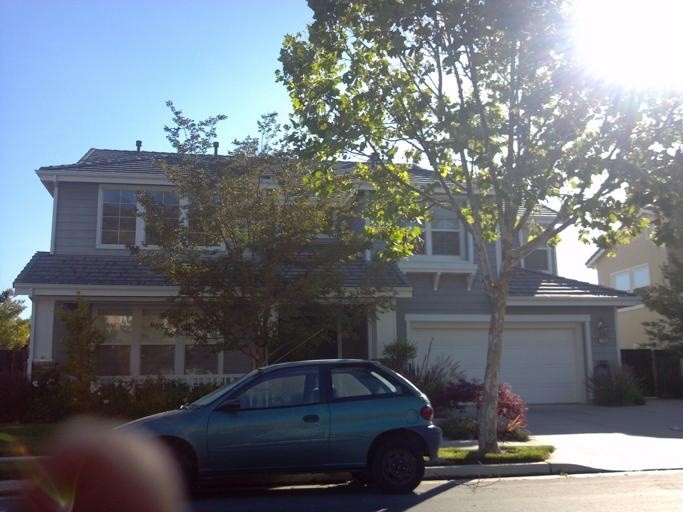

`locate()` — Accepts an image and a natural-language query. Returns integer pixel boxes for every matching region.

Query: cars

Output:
[105,357,441,494]
